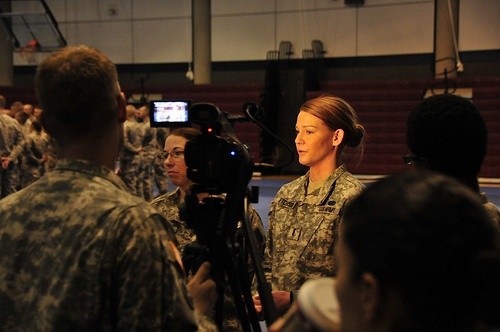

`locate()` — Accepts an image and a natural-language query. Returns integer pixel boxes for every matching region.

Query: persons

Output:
[0,45,267,332]
[332,94,500,332]
[253,94,367,332]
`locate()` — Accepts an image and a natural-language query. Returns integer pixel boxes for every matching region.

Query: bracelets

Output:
[290,291,294,303]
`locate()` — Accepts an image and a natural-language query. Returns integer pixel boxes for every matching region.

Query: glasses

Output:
[403,153,427,168]
[158,149,184,164]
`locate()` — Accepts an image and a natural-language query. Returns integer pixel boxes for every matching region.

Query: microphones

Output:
[242,100,266,123]
